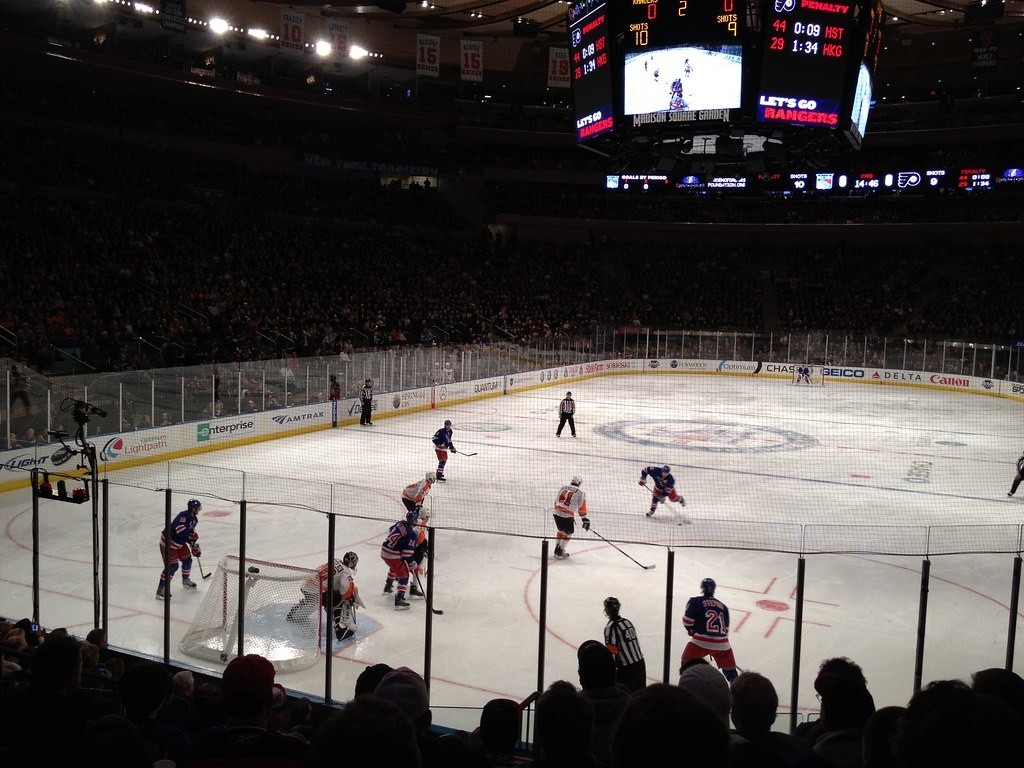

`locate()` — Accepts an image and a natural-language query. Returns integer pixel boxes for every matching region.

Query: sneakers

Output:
[436,475,446,483]
[182,577,197,589]
[408,582,425,600]
[155,587,172,601]
[382,587,393,596]
[553,546,569,559]
[394,593,411,610]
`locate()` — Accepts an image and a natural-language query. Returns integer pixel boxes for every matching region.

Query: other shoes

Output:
[679,496,685,507]
[646,510,654,517]
[1007,491,1013,497]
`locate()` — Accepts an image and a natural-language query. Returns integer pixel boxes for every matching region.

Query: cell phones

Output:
[32,624,39,631]
[98,663,106,675]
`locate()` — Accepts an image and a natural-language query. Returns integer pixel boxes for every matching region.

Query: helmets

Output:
[406,506,430,524]
[661,465,671,473]
[700,578,716,595]
[426,471,436,480]
[343,551,359,570]
[444,419,452,427]
[365,378,371,382]
[570,472,584,485]
[566,391,572,396]
[602,597,621,619]
[187,498,201,508]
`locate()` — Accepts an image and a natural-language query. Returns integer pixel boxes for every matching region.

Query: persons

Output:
[1006,450,1024,497]
[797,365,812,385]
[0,292,1024,768]
[642,53,693,110]
[682,579,739,682]
[409,509,429,595]
[155,499,202,598]
[330,375,340,400]
[359,378,373,427]
[432,420,456,480]
[556,392,577,437]
[639,465,685,517]
[381,511,422,609]
[605,597,647,690]
[402,473,437,521]
[286,551,358,641]
[553,477,590,560]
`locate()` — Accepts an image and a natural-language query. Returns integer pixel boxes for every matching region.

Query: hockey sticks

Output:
[447,447,477,457]
[416,573,443,614]
[588,527,656,569]
[197,556,212,580]
[644,484,693,525]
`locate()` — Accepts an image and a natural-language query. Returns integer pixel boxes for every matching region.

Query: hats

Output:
[678,657,731,717]
[372,671,427,723]
[576,639,615,689]
[222,655,276,704]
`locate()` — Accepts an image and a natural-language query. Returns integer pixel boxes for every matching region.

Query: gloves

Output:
[449,446,456,453]
[638,479,647,486]
[582,518,590,532]
[660,497,666,504]
[189,532,202,558]
[409,561,419,575]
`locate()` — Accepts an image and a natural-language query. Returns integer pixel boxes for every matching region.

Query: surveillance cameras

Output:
[91,407,107,418]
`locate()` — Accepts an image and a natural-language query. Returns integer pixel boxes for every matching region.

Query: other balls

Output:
[678,524,682,526]
